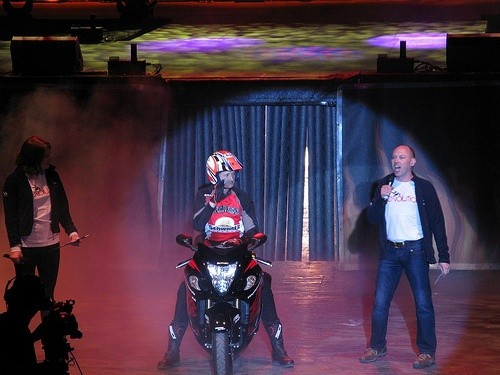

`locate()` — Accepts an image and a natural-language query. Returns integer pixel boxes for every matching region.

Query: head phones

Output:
[4,274,55,312]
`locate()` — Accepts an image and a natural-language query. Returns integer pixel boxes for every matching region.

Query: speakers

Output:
[10,36,84,75]
[446,33,500,71]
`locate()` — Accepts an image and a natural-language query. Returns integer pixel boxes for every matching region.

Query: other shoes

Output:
[358,348,388,363]
[413,354,436,369]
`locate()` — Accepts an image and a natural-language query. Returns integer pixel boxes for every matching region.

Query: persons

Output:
[359,145,451,369]
[2,136,81,352]
[0,273,71,375]
[158,151,294,369]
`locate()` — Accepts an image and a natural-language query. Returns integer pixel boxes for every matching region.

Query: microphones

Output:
[3,254,29,264]
[380,176,394,207]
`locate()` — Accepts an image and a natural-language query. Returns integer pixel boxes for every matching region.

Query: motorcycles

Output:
[175,233,273,375]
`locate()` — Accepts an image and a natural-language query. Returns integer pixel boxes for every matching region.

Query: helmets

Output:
[206,150,243,185]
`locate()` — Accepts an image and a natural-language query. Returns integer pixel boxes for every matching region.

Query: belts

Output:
[387,240,411,249]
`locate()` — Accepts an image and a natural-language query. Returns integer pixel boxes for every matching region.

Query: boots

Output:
[266,318,296,368]
[157,324,186,369]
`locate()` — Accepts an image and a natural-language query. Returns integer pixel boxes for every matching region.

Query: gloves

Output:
[254,233,266,242]
[209,180,232,203]
